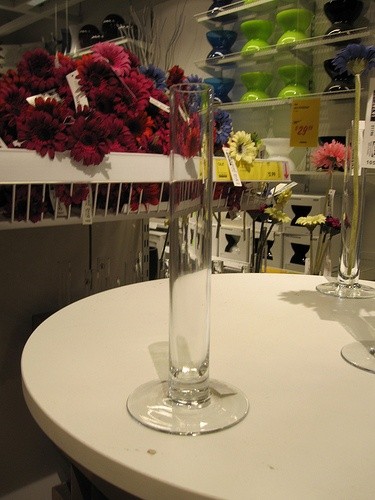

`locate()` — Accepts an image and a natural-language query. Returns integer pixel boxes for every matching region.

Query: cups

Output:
[315,130,374,299]
[340,337,375,373]
[126,84,249,436]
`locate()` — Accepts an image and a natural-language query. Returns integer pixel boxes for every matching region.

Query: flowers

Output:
[0,42,269,222]
[253,140,352,273]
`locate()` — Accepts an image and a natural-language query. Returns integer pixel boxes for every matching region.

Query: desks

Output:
[19,268,375,500]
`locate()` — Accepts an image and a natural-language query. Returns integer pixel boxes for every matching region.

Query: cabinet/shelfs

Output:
[194,0,375,272]
[0,0,153,67]
